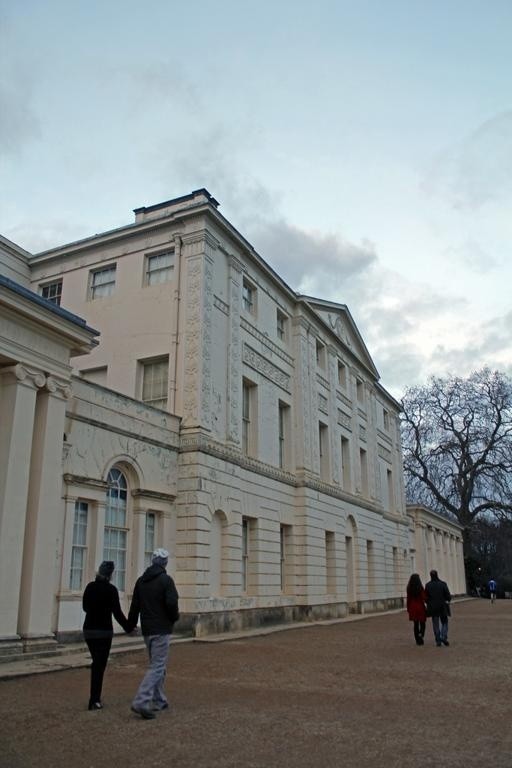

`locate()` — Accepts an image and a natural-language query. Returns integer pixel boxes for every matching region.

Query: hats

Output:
[97,559,117,579]
[150,547,170,568]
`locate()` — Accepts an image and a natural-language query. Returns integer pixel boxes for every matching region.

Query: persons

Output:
[469,584,487,598]
[80,559,126,711]
[424,570,454,645]
[487,576,498,603]
[125,547,179,720]
[404,572,429,645]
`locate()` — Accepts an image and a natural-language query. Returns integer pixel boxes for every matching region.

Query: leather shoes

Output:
[153,702,169,712]
[130,705,156,720]
[414,633,449,647]
[88,700,104,711]
